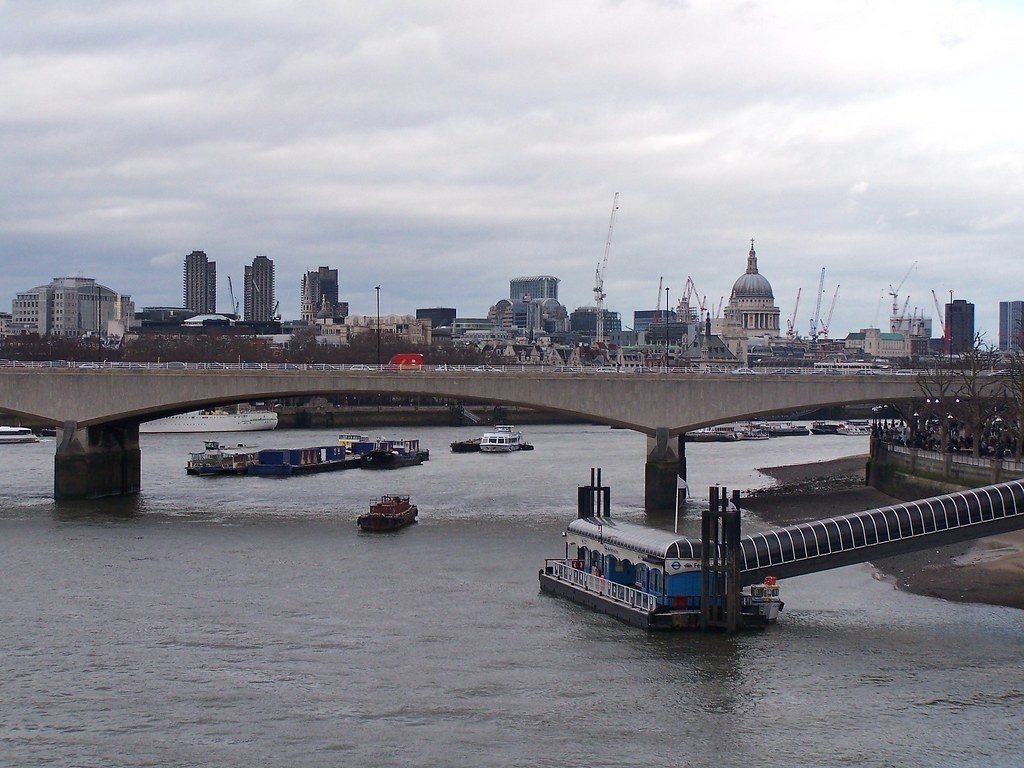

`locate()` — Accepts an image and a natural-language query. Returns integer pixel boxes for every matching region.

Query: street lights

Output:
[949,290,954,376]
[665,286,671,372]
[375,284,381,369]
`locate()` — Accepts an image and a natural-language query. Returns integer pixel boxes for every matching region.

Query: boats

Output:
[684,420,903,442]
[449,438,482,452]
[478,425,522,453]
[184,432,431,476]
[537,467,785,632]
[139,407,279,433]
[356,494,418,531]
[0,425,39,448]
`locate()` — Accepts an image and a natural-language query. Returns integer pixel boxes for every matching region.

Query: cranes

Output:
[652,276,663,322]
[931,288,946,333]
[887,260,919,335]
[785,287,803,338]
[591,191,623,342]
[807,267,826,340]
[677,275,724,321]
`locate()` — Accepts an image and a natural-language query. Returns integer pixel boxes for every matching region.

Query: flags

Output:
[678,477,690,498]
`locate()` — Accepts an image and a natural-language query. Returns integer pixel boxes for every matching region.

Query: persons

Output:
[870,423,1015,459]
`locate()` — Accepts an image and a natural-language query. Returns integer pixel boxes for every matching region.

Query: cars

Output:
[0,358,376,371]
[989,369,1021,376]
[434,364,502,373]
[553,366,917,376]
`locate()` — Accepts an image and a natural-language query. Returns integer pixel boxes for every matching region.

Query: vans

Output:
[382,354,424,371]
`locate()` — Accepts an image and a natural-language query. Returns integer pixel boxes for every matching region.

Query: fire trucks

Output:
[818,284,840,339]
